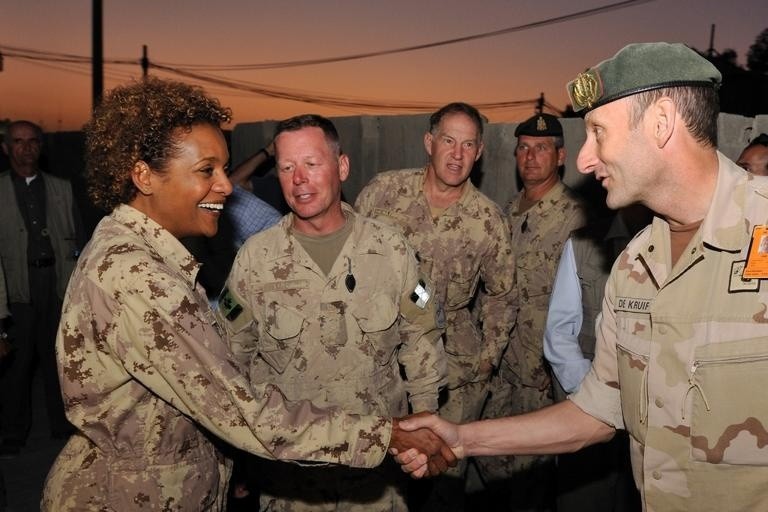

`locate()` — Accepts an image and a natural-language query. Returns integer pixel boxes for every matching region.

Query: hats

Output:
[514,113,564,137]
[566,42,720,113]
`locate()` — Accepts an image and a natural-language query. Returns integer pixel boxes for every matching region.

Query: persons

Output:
[386,38,768,512]
[732,132,768,176]
[37,76,459,512]
[1,102,655,512]
